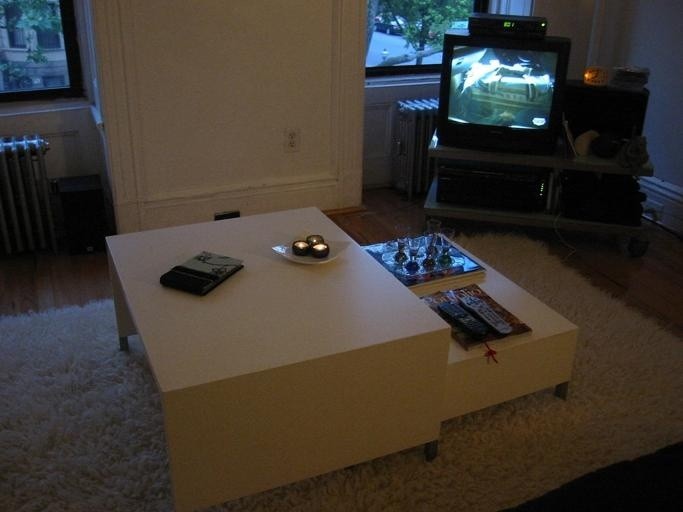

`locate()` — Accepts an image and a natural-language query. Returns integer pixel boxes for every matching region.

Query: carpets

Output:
[1,230,683,512]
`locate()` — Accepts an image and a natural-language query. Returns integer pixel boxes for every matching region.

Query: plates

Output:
[271,240,352,265]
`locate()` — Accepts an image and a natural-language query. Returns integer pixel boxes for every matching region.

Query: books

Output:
[159,250,242,296]
[608,64,650,92]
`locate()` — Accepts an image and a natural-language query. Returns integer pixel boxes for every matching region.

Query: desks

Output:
[359,231,582,423]
[103,201,451,512]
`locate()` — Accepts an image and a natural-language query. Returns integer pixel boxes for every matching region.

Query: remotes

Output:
[461,295,513,335]
[437,301,488,341]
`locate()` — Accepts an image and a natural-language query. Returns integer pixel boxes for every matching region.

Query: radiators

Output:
[0,137,56,255]
[391,100,440,201]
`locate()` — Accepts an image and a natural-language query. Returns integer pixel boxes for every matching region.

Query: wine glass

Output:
[396,219,455,269]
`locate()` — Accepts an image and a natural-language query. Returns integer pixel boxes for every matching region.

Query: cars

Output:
[373,12,441,40]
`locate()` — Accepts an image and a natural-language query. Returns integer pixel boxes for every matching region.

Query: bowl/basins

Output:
[610,65,649,93]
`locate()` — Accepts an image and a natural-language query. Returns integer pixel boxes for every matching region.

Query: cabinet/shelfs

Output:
[55,173,111,255]
[420,129,655,261]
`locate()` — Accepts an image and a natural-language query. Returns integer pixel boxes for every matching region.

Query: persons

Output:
[381,49,389,62]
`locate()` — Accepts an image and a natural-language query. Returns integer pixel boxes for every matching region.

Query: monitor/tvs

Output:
[436,28,572,155]
